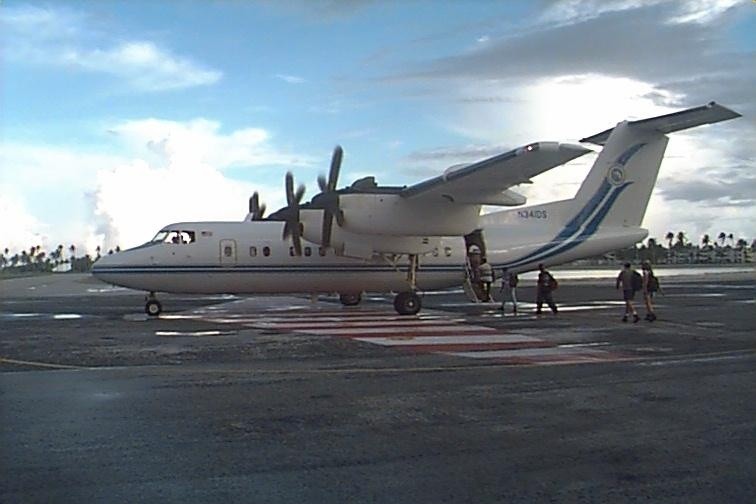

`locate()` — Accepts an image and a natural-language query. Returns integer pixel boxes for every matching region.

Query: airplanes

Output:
[88,96,745,321]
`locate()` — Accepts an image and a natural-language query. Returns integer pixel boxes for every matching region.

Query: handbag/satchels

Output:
[549,279,558,291]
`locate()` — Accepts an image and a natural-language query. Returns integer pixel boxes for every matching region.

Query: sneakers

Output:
[645,314,657,322]
[498,306,516,311]
[622,316,627,321]
[482,297,490,301]
[633,317,640,323]
[536,309,557,314]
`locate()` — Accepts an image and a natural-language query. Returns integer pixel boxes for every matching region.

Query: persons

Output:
[172,236,177,243]
[498,266,518,311]
[468,243,481,269]
[616,262,641,322]
[474,255,493,302]
[176,234,187,243]
[641,262,657,322]
[535,263,558,313]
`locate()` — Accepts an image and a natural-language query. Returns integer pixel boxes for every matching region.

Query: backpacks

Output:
[632,271,643,292]
[648,274,658,293]
[509,272,516,288]
